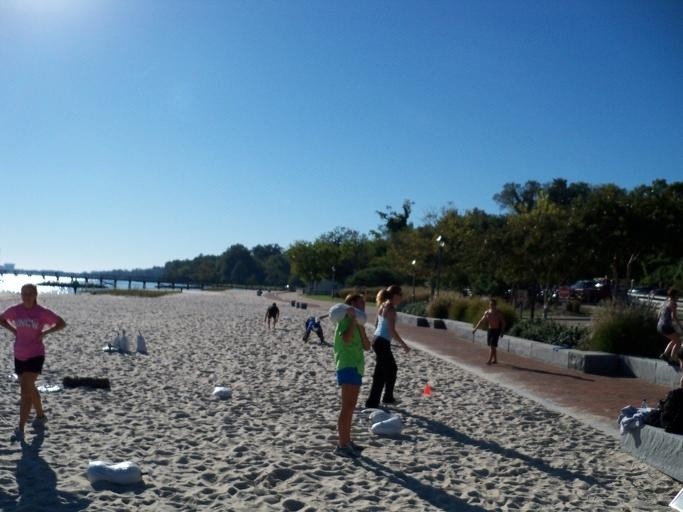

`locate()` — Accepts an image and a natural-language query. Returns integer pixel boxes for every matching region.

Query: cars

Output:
[536,277,612,304]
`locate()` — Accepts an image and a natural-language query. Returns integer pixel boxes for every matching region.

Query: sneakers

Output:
[31,415,48,427]
[333,441,365,458]
[9,427,24,440]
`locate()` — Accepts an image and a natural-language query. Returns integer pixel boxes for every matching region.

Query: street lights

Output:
[435,234,446,297]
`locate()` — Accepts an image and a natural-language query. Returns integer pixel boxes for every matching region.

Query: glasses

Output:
[396,293,404,296]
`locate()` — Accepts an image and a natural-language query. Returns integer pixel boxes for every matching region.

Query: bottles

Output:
[641,399,647,408]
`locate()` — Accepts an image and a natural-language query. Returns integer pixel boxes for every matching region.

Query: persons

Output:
[265,302,279,330]
[657,286,682,366]
[0,284,67,438]
[333,293,371,457]
[472,299,506,364]
[72,280,79,294]
[364,285,410,409]
[302,316,324,345]
